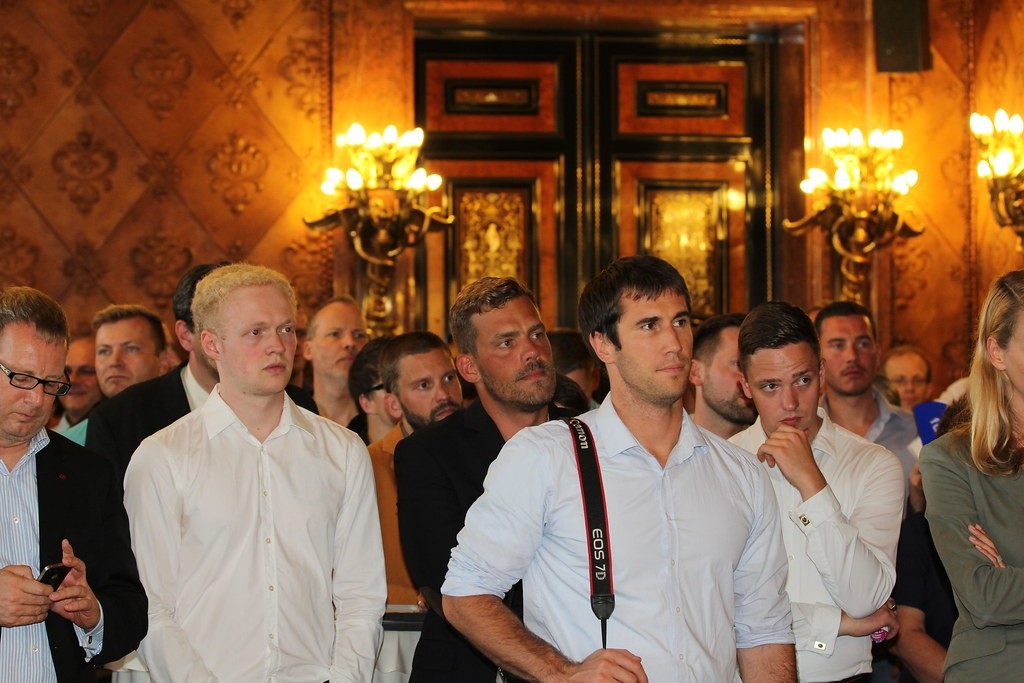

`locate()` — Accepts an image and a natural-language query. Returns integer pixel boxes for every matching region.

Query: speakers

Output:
[873,0,932,72]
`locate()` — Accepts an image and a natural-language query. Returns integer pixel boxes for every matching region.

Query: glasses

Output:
[0,363,72,396]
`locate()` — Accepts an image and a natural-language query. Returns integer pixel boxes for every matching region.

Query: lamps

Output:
[301,122,455,342]
[969,109,1023,255]
[782,127,925,306]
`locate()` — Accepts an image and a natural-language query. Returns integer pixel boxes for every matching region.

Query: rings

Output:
[997,554,1003,562]
[890,603,897,611]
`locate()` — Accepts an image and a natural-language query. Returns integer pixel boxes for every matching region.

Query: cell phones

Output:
[36,563,74,592]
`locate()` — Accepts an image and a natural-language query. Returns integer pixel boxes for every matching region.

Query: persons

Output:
[0,252,1024,683]
